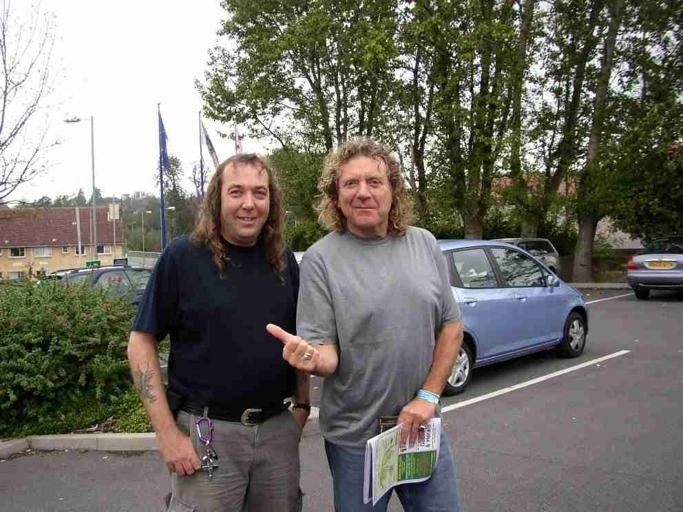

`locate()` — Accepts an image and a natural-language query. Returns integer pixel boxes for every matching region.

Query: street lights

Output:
[158,206,177,252]
[140,209,152,268]
[63,116,98,260]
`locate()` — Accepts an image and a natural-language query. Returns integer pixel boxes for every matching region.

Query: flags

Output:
[159,112,168,169]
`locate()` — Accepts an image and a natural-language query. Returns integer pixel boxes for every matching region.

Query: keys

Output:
[200,444,218,479]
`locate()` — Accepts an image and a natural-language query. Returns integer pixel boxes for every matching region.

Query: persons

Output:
[266,139,465,512]
[127,155,310,512]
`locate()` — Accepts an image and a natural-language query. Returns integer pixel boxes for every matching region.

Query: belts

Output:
[180,399,293,427]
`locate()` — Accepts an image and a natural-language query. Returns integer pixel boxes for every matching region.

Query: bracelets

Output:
[416,389,441,405]
[292,401,311,415]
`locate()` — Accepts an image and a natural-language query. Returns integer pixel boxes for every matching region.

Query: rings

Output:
[418,425,427,432]
[304,352,311,360]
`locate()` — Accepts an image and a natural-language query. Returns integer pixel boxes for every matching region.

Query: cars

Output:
[624,236,683,300]
